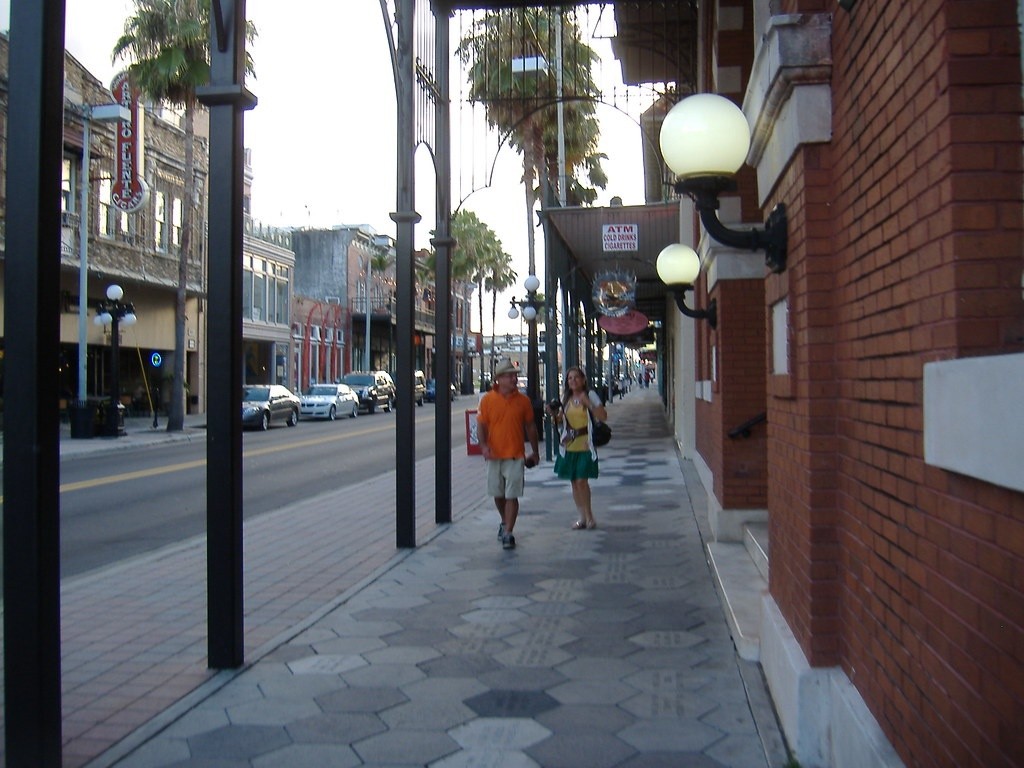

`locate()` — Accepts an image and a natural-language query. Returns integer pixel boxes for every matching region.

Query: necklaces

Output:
[572,395,583,404]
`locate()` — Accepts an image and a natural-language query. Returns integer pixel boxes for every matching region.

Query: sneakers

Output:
[498,522,512,541]
[502,534,515,549]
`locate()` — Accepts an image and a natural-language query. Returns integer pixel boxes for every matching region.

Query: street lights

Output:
[92,285,138,425]
[78,105,131,409]
[508,275,547,442]
[364,237,394,372]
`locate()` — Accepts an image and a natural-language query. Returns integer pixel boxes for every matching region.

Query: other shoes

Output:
[586,520,596,528]
[571,520,586,529]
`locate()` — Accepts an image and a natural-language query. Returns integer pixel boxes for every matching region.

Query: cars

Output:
[516,376,544,400]
[243,384,302,431]
[423,378,457,401]
[298,383,359,421]
[478,371,491,383]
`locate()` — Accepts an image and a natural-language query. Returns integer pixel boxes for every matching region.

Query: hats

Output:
[493,359,521,379]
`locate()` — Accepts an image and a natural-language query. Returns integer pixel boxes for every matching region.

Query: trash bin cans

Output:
[100,398,127,435]
[466,410,482,455]
[68,399,96,438]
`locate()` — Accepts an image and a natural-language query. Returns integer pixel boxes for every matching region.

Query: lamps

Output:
[660,94,787,275]
[656,243,718,329]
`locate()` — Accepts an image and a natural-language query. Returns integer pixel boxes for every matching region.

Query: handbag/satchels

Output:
[584,387,612,447]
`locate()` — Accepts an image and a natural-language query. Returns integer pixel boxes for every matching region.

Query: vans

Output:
[389,370,427,406]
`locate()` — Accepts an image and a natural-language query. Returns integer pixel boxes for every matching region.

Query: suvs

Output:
[335,370,396,414]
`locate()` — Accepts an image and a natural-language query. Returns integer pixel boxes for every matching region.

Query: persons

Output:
[635,368,658,390]
[546,365,608,530]
[476,359,541,550]
